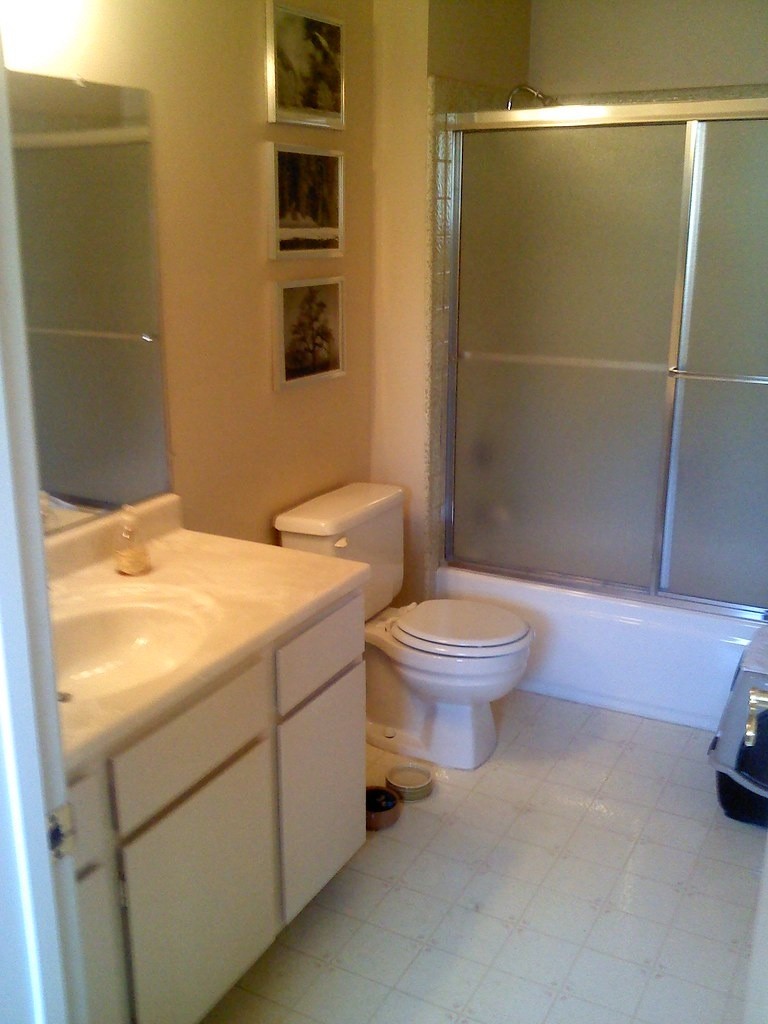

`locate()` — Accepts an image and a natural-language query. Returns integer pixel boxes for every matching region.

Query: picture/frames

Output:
[270,274,348,394]
[265,1,345,131]
[264,141,345,261]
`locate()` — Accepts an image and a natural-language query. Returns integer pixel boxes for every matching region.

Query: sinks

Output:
[42,582,230,703]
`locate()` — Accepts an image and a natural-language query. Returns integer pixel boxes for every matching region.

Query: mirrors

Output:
[7,69,173,538]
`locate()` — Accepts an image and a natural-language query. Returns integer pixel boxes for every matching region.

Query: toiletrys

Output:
[113,505,153,574]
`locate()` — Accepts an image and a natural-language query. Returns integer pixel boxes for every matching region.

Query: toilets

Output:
[272,481,534,770]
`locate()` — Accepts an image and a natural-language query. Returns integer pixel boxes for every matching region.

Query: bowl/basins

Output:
[366,786,402,831]
[385,763,433,802]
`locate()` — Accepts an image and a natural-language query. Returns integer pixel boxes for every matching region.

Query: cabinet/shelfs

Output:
[65,586,366,1024]
